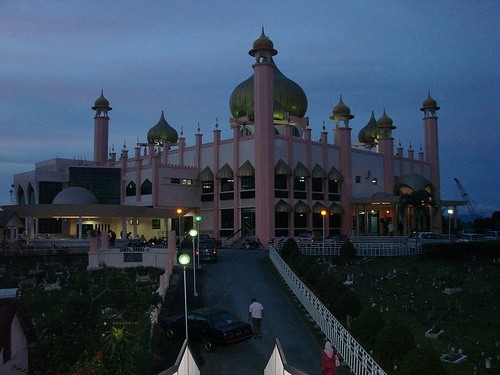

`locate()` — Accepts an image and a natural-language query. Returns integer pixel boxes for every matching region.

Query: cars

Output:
[298,231,353,254]
[160,306,255,354]
[407,231,452,253]
[456,231,500,242]
[181,234,218,263]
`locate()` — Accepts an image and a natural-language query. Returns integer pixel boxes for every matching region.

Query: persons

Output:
[321,341,340,375]
[248,298,265,339]
[388,222,394,238]
[87,229,168,247]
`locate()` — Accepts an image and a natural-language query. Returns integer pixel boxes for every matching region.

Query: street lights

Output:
[176,208,183,243]
[321,210,327,255]
[447,208,454,239]
[189,228,198,297]
[178,253,191,340]
[195,215,202,269]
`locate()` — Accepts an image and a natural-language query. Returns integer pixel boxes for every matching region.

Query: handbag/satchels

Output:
[335,358,340,367]
